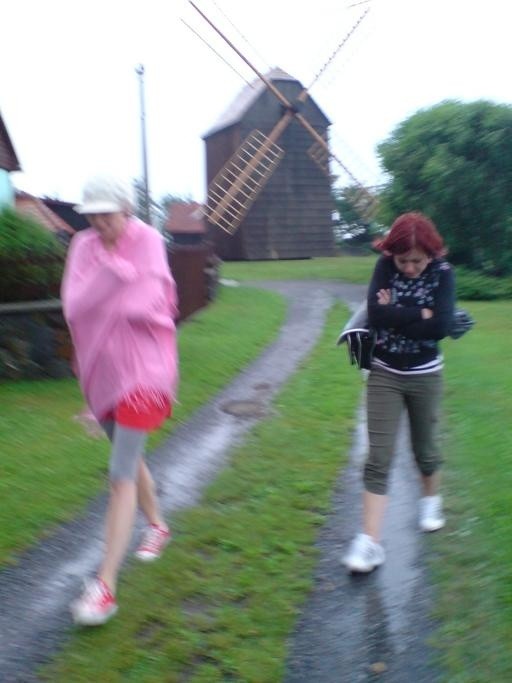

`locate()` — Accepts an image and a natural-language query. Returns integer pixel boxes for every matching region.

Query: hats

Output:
[72,179,133,216]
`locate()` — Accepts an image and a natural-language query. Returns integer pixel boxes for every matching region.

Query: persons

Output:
[59,174,180,628]
[341,210,456,573]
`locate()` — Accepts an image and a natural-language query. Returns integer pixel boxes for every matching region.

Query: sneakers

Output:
[415,494,446,533]
[341,534,385,575]
[71,575,120,628]
[136,521,173,562]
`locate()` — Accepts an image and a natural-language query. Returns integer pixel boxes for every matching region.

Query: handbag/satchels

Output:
[337,301,373,370]
